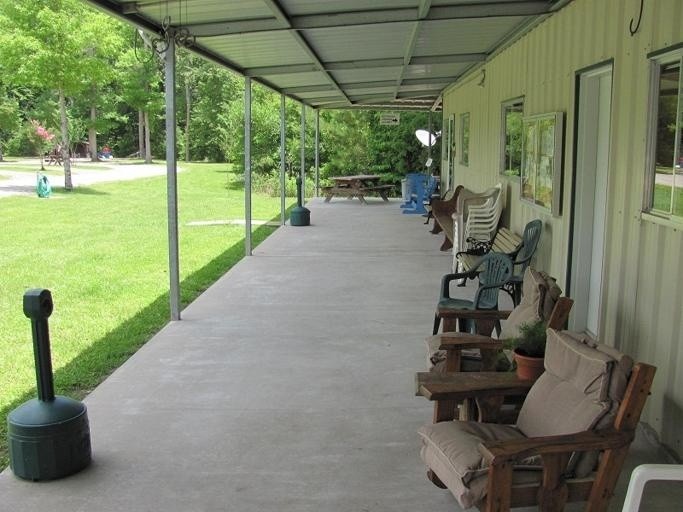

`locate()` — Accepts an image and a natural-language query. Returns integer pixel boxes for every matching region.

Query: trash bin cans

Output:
[401,179,408,200]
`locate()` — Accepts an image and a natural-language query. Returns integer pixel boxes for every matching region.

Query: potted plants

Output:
[503,318,553,383]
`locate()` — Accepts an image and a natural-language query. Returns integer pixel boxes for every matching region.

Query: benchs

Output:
[322,183,396,206]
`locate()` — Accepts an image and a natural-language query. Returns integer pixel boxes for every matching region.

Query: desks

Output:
[329,175,380,200]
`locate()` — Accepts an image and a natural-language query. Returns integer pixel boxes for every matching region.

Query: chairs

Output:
[414,326,659,512]
[403,173,505,283]
[437,219,543,336]
[425,264,575,373]
